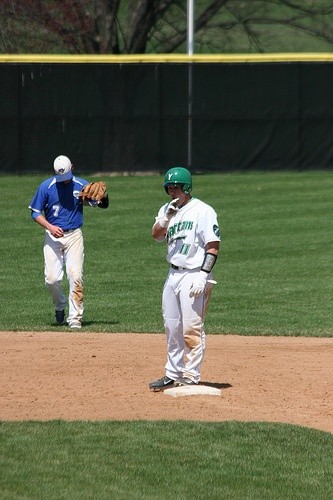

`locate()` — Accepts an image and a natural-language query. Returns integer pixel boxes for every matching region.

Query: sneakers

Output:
[173,377,195,387]
[149,376,174,391]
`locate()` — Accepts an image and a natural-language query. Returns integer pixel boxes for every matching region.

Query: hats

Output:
[53,154,73,183]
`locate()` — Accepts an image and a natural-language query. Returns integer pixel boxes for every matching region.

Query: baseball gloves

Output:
[78,182,107,202]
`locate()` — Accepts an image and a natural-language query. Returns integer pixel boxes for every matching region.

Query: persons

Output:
[148,167,220,392]
[28,155,109,328]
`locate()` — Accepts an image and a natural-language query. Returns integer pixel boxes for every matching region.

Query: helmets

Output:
[163,167,193,195]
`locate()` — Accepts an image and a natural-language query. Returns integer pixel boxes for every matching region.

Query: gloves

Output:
[158,198,180,229]
[188,270,209,298]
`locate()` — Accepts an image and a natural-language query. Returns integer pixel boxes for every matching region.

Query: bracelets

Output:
[201,253,218,274]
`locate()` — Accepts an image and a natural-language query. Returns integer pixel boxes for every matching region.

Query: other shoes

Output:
[70,322,82,329]
[54,309,65,324]
